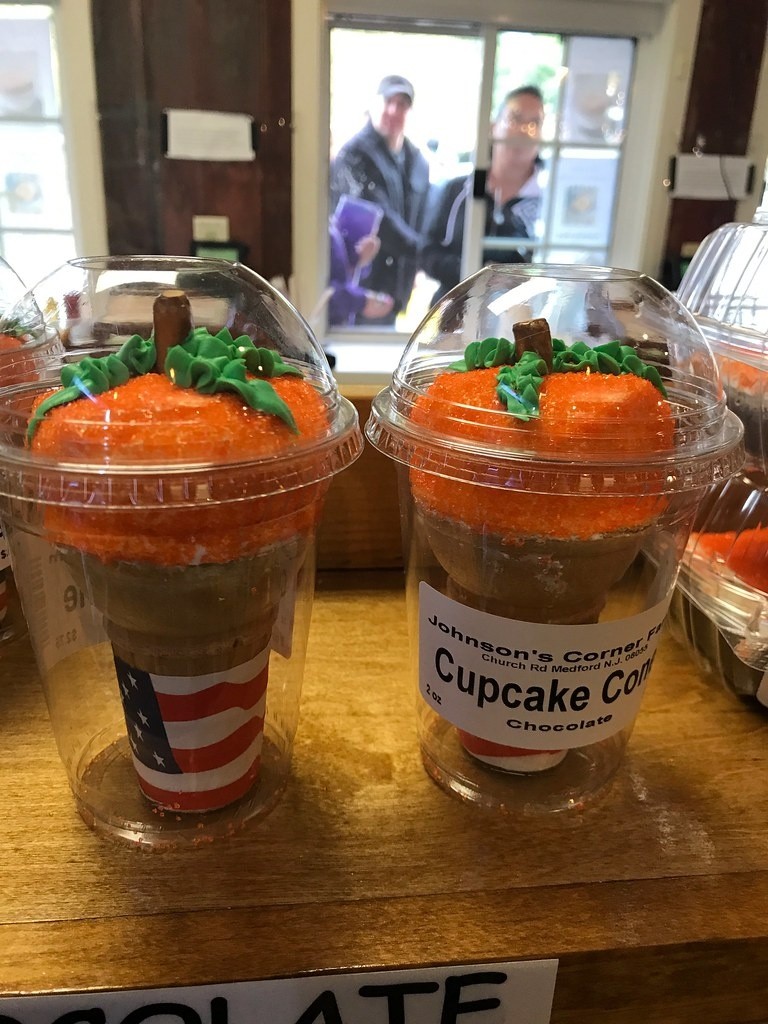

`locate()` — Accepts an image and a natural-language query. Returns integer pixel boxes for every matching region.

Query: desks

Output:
[0,586,768,1024]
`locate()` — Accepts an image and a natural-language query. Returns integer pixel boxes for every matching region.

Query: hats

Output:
[377,75,415,105]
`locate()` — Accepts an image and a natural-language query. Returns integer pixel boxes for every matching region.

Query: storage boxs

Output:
[637,222,768,707]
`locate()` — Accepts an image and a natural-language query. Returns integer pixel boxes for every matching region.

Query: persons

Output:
[327,75,549,329]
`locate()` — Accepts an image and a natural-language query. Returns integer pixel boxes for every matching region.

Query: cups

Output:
[1,253,364,855]
[364,258,744,823]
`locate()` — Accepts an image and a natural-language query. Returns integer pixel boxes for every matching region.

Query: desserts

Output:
[403,316,674,774]
[665,339,768,646]
[22,289,330,815]
